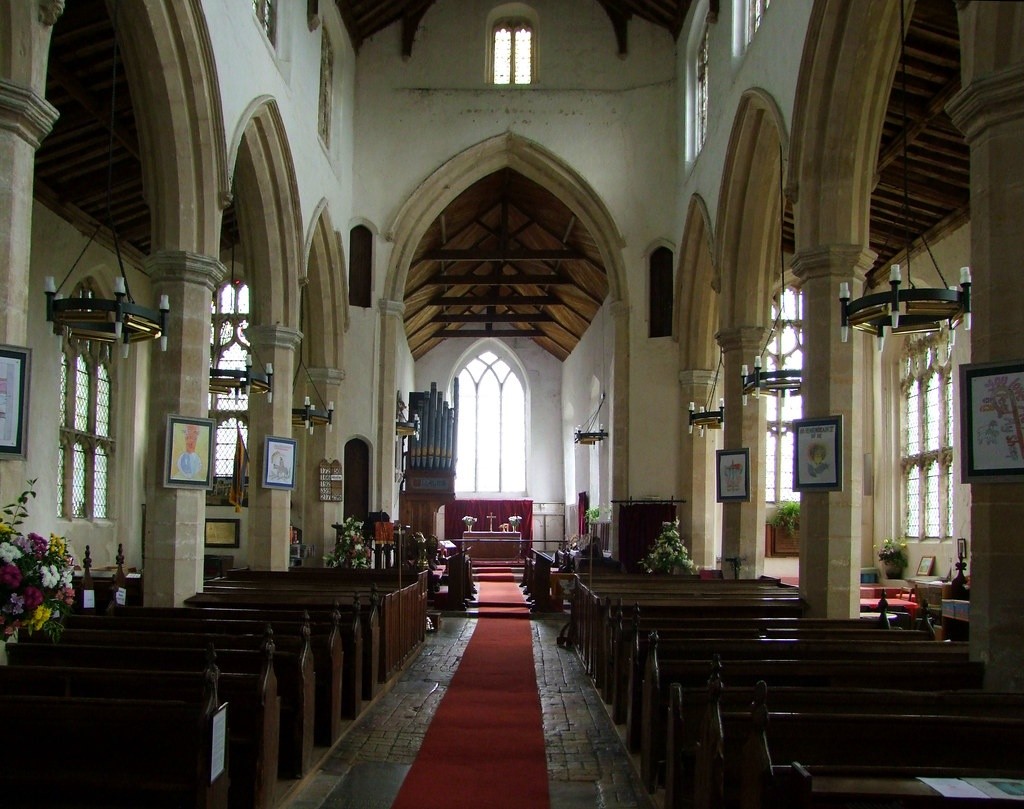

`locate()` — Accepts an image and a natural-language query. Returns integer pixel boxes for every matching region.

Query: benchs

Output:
[557,567,1024,809]
[0,568,433,809]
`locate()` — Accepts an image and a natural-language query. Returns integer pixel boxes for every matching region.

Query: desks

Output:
[461,531,524,560]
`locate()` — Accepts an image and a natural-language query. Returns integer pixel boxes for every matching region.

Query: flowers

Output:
[508,515,522,526]
[640,512,702,578]
[461,515,478,526]
[0,476,78,649]
[322,511,374,569]
[870,536,910,569]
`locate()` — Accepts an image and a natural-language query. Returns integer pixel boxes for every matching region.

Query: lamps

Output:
[43,0,172,361]
[210,209,275,407]
[396,387,423,443]
[737,142,803,406]
[687,346,724,437]
[574,393,608,446]
[835,0,970,353]
[292,287,336,438]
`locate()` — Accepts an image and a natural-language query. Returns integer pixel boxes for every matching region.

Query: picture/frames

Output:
[206,701,230,788]
[259,431,298,493]
[789,413,846,494]
[957,358,1024,484]
[916,553,935,576]
[204,516,241,550]
[714,447,752,504]
[0,342,34,462]
[161,412,217,493]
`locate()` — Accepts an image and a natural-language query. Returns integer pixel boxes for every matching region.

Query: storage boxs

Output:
[860,567,881,584]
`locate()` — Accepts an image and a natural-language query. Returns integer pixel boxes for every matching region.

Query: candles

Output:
[959,542,964,553]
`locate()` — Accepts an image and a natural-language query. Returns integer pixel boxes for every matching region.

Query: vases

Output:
[883,562,903,580]
[512,525,517,532]
[467,525,473,532]
[668,565,684,576]
